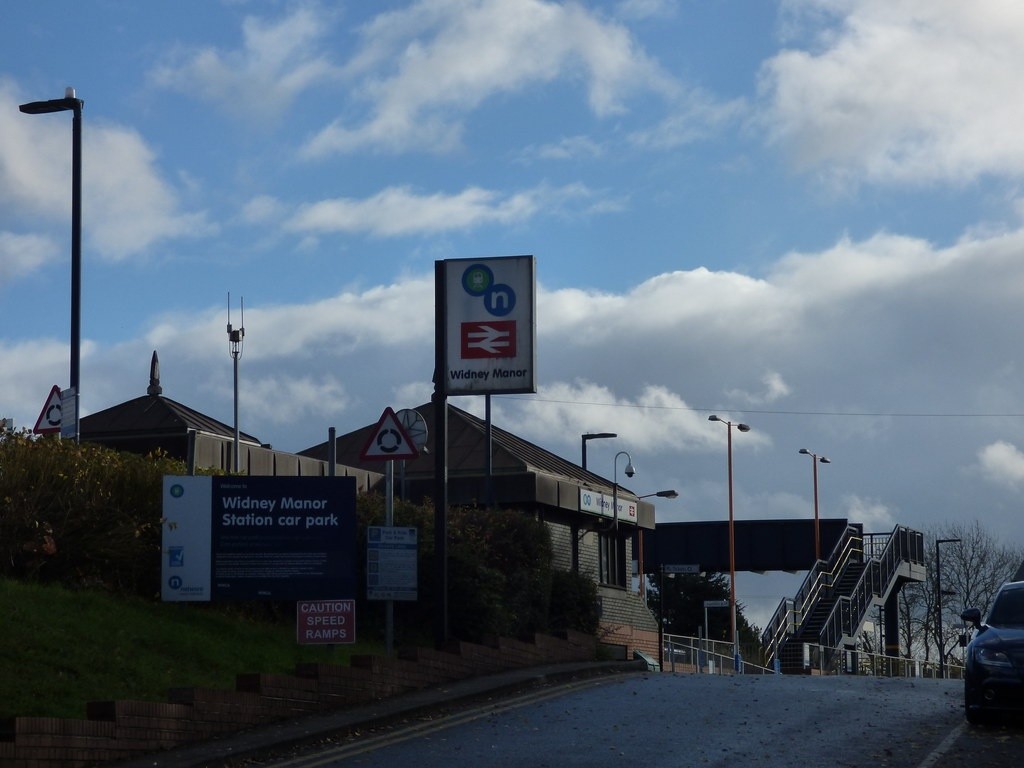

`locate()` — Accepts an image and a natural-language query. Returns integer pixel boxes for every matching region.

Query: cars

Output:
[960,580,1024,727]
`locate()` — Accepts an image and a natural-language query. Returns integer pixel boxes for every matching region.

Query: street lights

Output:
[636,490,679,596]
[612,450,636,586]
[934,538,964,677]
[19,96,80,446]
[799,448,830,588]
[582,432,617,472]
[709,414,750,673]
